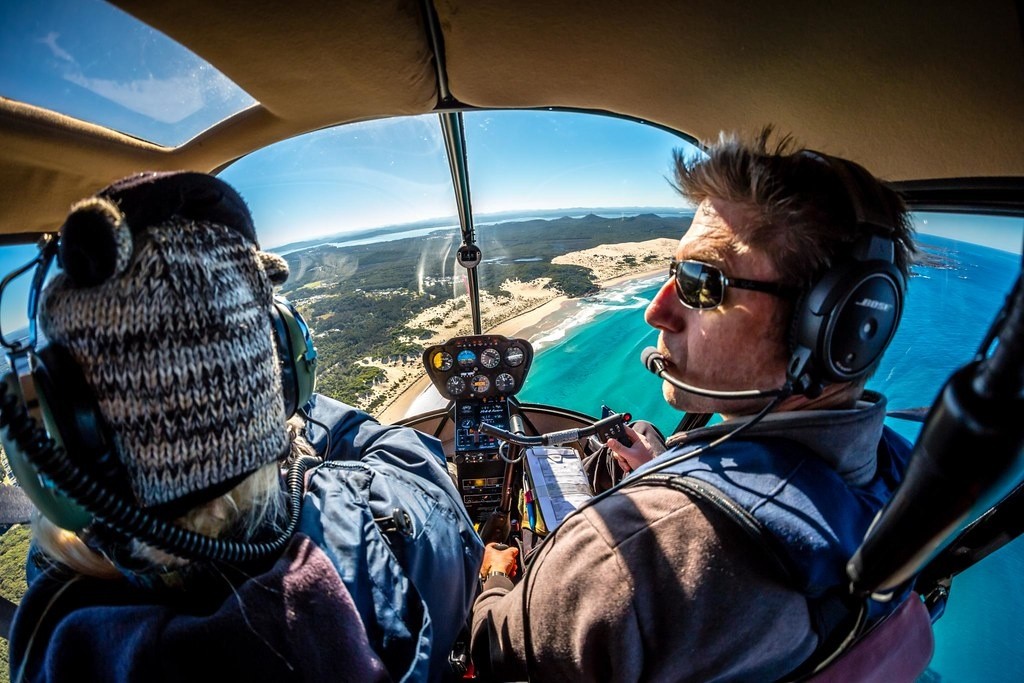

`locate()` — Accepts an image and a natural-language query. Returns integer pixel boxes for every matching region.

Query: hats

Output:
[38,199,290,508]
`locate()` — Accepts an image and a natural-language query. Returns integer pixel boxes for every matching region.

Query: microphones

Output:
[642,347,782,400]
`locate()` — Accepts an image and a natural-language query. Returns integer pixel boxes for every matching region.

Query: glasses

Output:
[670,257,782,310]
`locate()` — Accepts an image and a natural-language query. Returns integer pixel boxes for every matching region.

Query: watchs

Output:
[478,572,509,592]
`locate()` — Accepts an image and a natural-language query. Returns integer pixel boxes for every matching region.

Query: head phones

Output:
[781,149,904,400]
[0,228,319,539]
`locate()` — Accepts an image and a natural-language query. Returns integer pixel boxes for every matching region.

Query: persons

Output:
[0,169,486,683]
[470,122,951,683]
